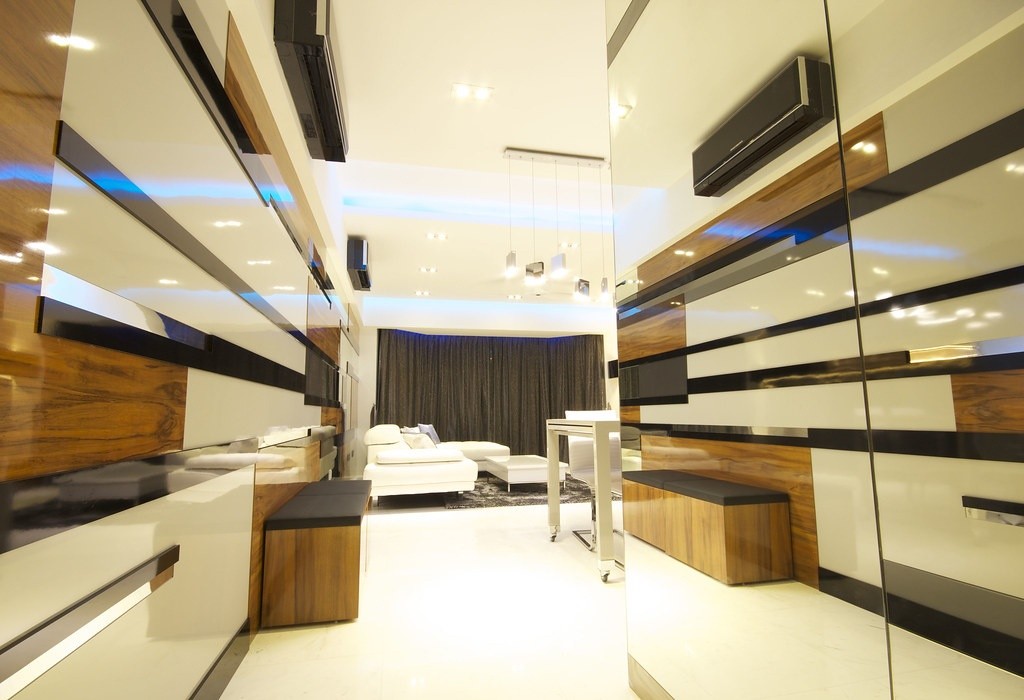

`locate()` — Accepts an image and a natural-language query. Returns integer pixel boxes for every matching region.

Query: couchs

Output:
[363,423,511,501]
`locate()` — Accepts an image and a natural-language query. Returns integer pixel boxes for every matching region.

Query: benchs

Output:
[262,478,376,628]
[617,470,797,586]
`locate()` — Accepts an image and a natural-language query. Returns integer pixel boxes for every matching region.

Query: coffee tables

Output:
[484,455,569,494]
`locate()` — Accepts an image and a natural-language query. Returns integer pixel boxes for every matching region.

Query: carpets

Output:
[573,530,624,575]
[441,477,621,508]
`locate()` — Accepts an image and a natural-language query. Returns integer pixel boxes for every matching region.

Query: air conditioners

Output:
[346,236,375,290]
[273,1,349,163]
[692,55,836,200]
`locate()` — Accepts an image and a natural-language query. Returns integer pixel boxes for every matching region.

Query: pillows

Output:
[419,424,441,445]
[402,433,436,449]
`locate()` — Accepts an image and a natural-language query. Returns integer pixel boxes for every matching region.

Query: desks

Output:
[546,420,621,582]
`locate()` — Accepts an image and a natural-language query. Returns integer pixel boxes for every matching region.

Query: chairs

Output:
[565,410,622,551]
